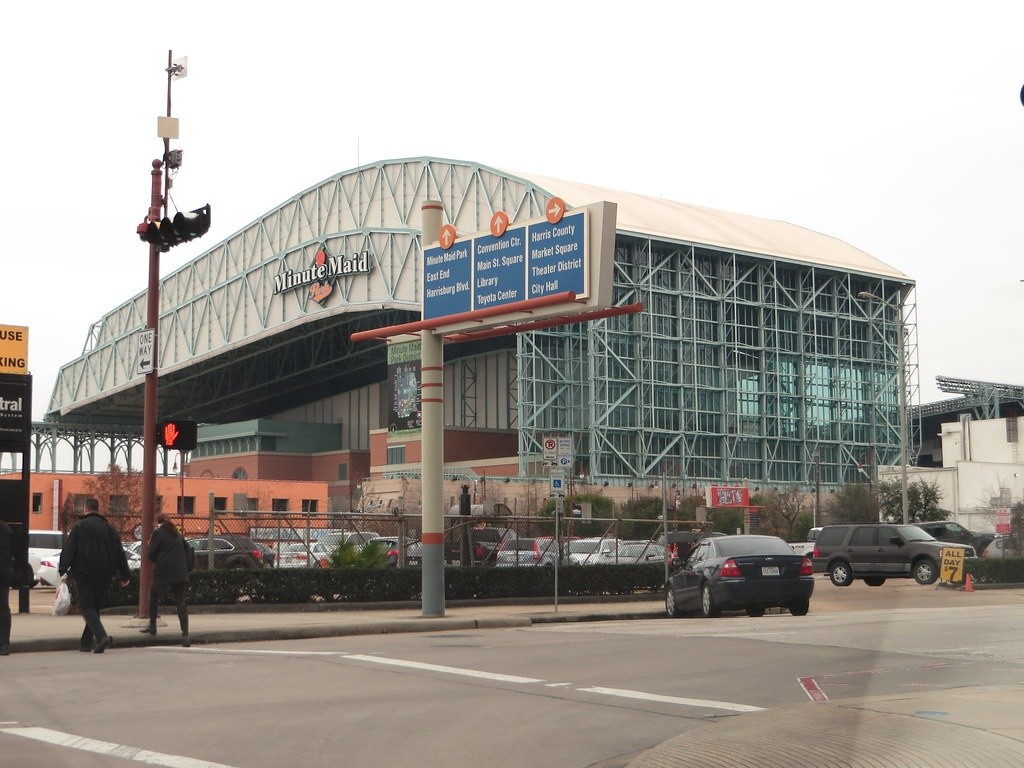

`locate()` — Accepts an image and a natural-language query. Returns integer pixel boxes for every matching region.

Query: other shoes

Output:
[78,645,92,652]
[93,638,111,653]
[0,644,11,655]
[139,628,157,635]
[182,634,190,647]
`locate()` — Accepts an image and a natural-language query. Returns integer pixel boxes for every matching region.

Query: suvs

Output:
[655,531,729,566]
[186,536,264,571]
[813,523,977,587]
[318,532,380,555]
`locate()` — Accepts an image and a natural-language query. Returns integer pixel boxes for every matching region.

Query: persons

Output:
[0,519,16,655]
[58,498,131,653]
[139,513,190,647]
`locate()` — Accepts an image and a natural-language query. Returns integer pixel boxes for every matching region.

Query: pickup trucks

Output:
[788,527,826,560]
[445,528,519,567]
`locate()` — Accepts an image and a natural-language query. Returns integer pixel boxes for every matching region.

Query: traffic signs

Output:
[137,327,156,375]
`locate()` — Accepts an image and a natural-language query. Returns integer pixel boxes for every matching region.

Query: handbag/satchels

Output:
[184,542,195,571]
[52,582,71,616]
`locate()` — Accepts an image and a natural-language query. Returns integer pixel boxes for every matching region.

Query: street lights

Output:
[857,291,908,525]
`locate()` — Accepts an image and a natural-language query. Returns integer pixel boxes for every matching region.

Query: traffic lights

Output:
[160,418,198,451]
[145,203,211,251]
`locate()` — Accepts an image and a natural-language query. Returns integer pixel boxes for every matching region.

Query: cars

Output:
[8,530,70,589]
[366,536,422,568]
[495,536,675,567]
[38,546,142,589]
[913,520,1024,560]
[256,543,275,568]
[664,534,814,618]
[273,542,339,569]
[122,540,142,554]
